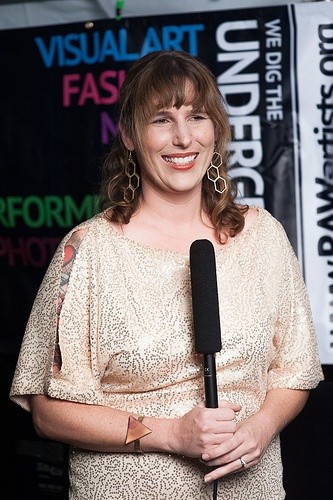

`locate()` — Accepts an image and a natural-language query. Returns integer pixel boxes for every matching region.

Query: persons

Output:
[10,49,325,500]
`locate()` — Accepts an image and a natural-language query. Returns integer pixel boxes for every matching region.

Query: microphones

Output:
[191,239,222,409]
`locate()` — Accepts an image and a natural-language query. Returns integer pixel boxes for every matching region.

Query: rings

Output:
[239,457,248,468]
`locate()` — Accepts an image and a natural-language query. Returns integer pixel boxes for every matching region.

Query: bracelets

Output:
[125,414,152,454]
